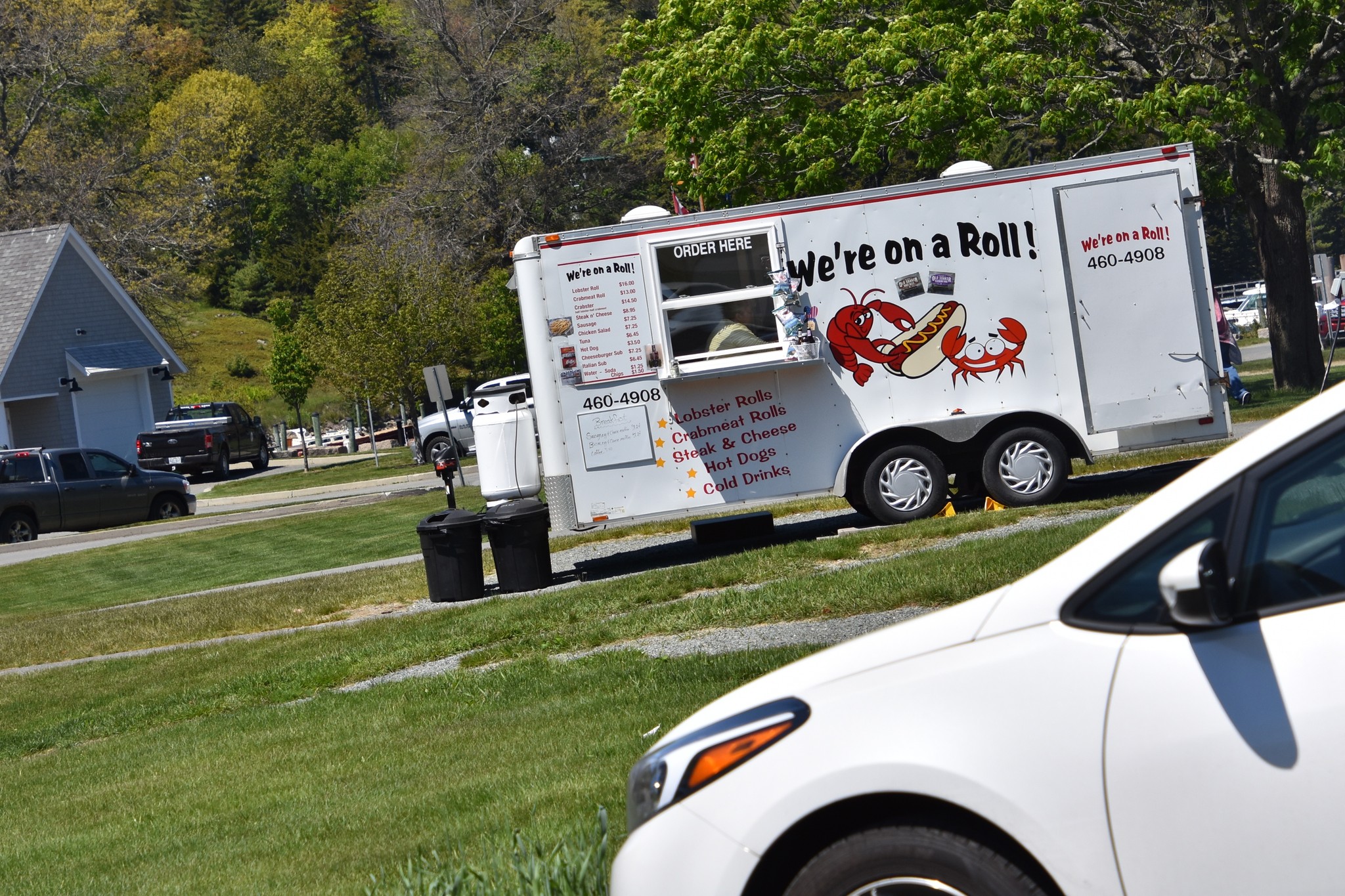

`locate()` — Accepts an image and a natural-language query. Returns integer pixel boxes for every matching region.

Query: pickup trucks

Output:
[136,401,269,481]
[0,447,198,545]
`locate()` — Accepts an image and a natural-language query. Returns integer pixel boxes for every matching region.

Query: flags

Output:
[688,142,696,169]
[672,192,689,216]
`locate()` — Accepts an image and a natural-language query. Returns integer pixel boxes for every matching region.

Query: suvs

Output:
[416,373,539,464]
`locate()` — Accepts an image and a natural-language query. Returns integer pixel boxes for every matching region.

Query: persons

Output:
[1212,286,1252,406]
[707,299,771,354]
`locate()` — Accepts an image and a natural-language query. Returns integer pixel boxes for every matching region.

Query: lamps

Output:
[59,377,83,394]
[152,366,175,382]
[76,328,86,336]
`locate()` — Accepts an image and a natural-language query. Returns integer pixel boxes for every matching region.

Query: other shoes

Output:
[1241,392,1252,405]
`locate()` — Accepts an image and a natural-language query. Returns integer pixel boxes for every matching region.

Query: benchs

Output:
[268,446,280,459]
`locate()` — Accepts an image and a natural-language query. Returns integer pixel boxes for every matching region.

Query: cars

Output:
[609,371,1345,896]
[1222,275,1345,350]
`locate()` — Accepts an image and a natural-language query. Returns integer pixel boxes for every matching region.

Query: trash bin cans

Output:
[417,508,485,602]
[482,498,553,593]
